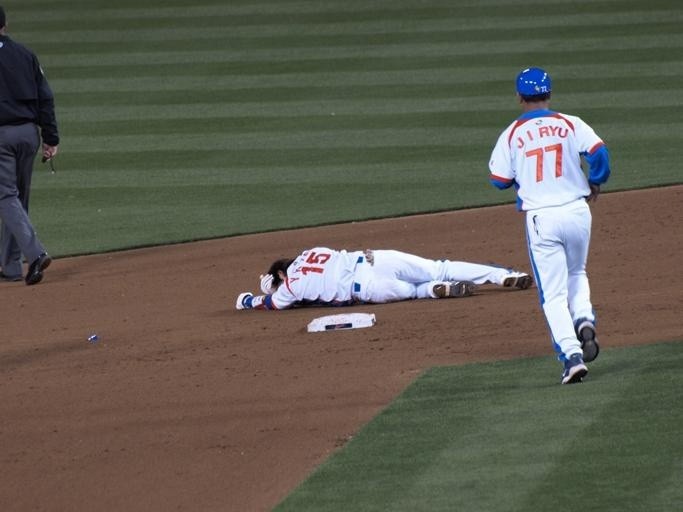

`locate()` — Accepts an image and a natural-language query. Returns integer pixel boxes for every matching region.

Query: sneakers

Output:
[431,281,476,298]
[25,251,51,285]
[562,356,587,382]
[0,271,23,281]
[577,319,599,362]
[502,271,531,289]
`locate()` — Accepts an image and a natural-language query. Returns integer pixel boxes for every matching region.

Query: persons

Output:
[0,6,61,287]
[235,245,532,311]
[487,66,611,386]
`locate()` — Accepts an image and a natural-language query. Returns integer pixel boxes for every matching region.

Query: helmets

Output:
[517,68,552,95]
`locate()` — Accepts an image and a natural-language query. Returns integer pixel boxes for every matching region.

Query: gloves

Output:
[259,274,276,295]
[235,293,253,311]
[586,177,599,202]
